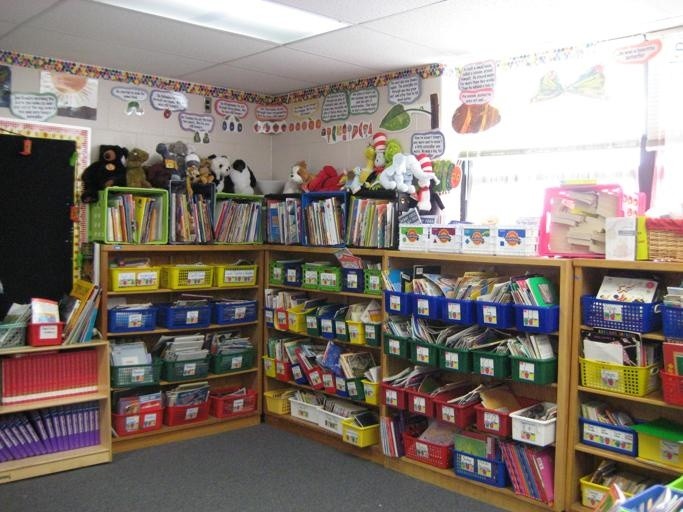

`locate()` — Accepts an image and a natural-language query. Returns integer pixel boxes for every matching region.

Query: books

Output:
[108,259,259,436]
[260,247,383,448]
[550,190,619,253]
[380,264,559,506]
[576,276,681,510]
[1,278,102,349]
[106,194,394,248]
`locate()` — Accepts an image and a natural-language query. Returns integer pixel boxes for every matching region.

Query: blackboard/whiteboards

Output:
[0,115,90,325]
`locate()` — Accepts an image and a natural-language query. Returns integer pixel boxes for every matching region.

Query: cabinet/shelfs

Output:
[260,243,385,466]
[83,242,262,453]
[380,251,572,512]
[0,339,112,484]
[566,244,683,512]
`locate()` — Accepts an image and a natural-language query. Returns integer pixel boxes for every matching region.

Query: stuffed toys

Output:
[283,132,440,211]
[83,140,256,203]
